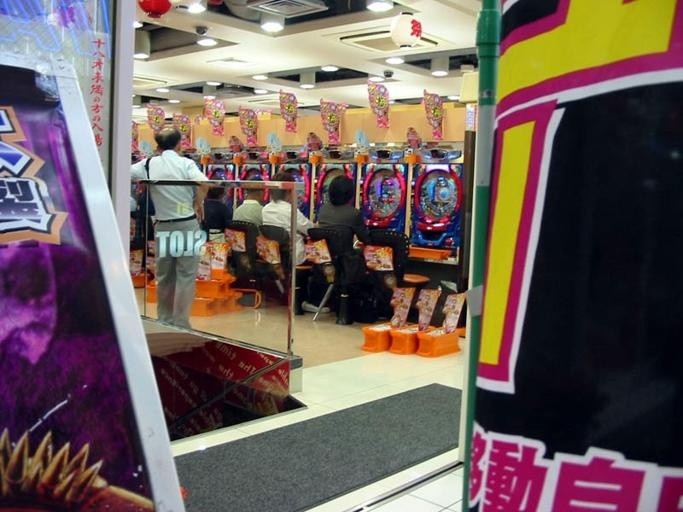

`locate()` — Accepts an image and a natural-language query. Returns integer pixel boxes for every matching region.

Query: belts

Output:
[155,213,198,223]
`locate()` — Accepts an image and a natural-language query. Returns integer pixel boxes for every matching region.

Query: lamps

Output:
[132,0,462,109]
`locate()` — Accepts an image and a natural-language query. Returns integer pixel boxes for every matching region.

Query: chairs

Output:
[130,211,431,326]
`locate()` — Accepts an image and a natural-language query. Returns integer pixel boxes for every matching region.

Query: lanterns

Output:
[138,0,172,18]
[388,9,424,48]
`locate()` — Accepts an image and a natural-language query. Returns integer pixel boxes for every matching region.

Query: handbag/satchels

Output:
[139,157,156,217]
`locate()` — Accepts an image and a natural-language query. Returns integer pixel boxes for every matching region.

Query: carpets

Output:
[173,382,462,511]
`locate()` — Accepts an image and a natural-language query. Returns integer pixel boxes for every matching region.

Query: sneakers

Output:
[301,300,331,314]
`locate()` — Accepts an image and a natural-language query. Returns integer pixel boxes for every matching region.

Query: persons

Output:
[131,124,209,332]
